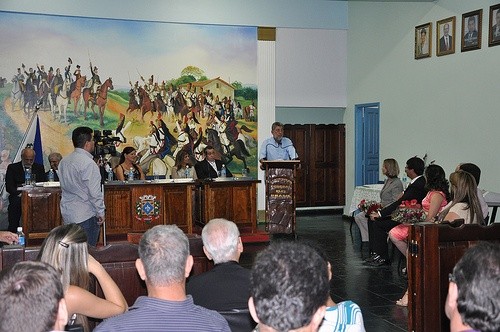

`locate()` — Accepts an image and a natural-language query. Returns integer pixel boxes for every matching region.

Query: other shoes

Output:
[365,253,382,262]
[368,257,391,267]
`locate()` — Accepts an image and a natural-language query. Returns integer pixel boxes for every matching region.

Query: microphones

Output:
[263,141,297,161]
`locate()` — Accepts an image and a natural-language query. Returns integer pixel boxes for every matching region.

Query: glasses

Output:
[25,159,33,163]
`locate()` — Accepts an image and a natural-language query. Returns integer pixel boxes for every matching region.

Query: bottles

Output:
[49,169,54,181]
[402,173,407,190]
[16,227,26,249]
[221,164,226,177]
[108,168,113,182]
[185,165,190,179]
[25,169,31,185]
[128,168,134,182]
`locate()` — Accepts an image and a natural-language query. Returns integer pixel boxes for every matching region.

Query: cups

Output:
[241,169,248,177]
[154,174,159,182]
[31,174,36,185]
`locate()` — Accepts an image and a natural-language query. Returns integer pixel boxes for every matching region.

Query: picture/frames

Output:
[436,16,456,56]
[488,3,500,47]
[461,9,483,52]
[414,21,432,59]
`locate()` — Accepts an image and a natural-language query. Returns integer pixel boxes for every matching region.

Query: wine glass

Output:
[217,170,220,178]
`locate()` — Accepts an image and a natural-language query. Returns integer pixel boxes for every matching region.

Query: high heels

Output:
[395,289,409,306]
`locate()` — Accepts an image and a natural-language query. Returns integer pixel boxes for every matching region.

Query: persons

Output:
[186,219,257,332]
[417,28,429,54]
[194,146,233,179]
[0,231,20,244]
[171,150,197,179]
[5,148,48,233]
[258,122,298,164]
[58,127,107,246]
[439,24,452,52]
[116,147,145,180]
[463,17,478,47]
[0,260,68,332]
[445,243,500,332]
[354,156,489,307]
[45,153,62,182]
[243,232,366,332]
[92,223,232,332]
[492,10,500,42]
[36,223,129,332]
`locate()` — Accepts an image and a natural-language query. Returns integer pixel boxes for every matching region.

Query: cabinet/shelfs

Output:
[16,178,261,246]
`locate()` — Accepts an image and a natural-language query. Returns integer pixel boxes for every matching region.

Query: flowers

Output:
[358,199,383,215]
[391,200,423,223]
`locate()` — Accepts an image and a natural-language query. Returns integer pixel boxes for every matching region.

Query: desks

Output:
[348,184,384,241]
